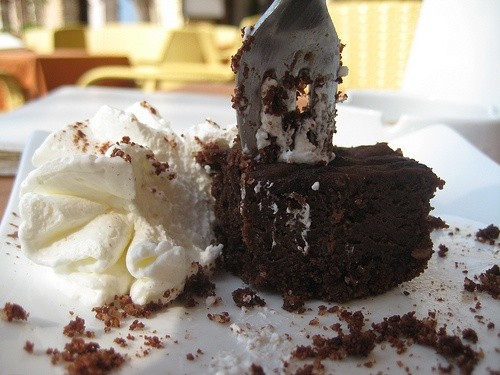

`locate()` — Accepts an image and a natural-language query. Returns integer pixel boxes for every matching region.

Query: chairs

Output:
[0,0,421,112]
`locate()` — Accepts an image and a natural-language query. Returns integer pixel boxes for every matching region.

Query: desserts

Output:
[19,1,443,308]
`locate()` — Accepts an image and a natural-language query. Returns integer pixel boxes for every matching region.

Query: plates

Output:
[0,83,500,375]
